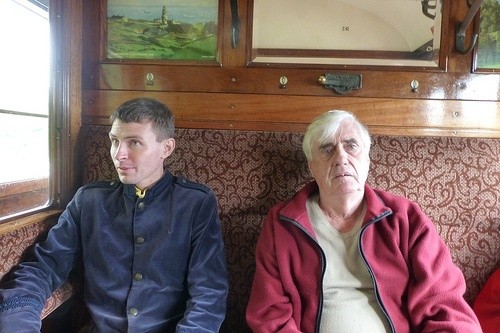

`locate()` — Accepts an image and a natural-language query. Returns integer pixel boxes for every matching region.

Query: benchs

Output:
[0,122,500,333]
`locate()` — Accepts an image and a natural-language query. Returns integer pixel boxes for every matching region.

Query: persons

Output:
[240,109,490,332]
[0,95,235,333]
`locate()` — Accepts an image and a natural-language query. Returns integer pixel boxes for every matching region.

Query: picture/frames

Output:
[245,0,452,73]
[98,0,225,68]
[470,0,500,74]
